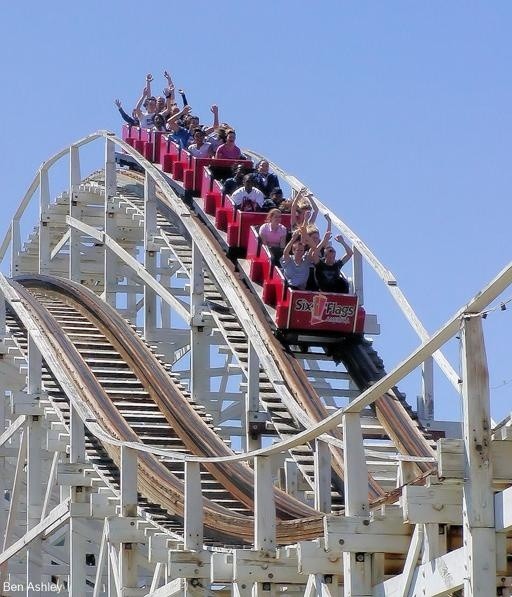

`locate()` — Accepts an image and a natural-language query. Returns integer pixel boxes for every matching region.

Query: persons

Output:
[115,64,355,295]
